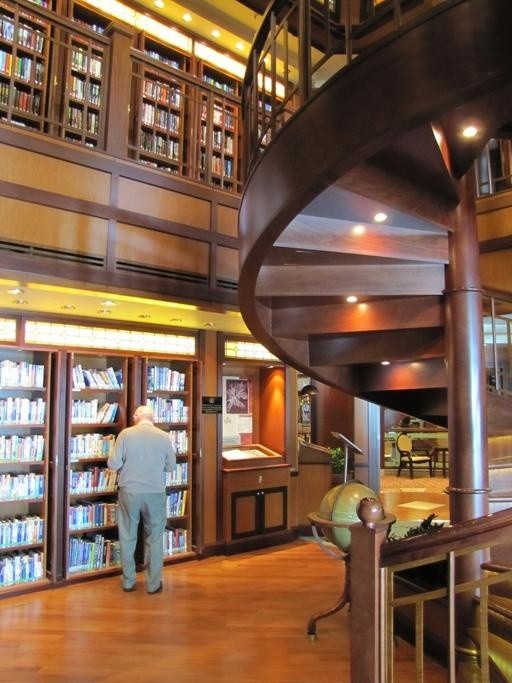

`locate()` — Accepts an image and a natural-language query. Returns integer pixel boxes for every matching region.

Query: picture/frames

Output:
[225,378,250,415]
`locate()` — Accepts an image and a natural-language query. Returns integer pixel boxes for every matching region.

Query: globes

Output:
[307,479,397,640]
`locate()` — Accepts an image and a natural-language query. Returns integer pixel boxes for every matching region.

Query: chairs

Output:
[395,434,433,480]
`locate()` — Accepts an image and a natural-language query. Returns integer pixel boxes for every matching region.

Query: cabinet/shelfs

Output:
[64,0,142,159]
[196,59,287,194]
[56,346,142,590]
[140,351,204,572]
[138,29,197,176]
[0,337,64,602]
[220,471,287,553]
[0,0,62,136]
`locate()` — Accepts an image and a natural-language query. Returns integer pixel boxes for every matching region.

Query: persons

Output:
[106,405,178,596]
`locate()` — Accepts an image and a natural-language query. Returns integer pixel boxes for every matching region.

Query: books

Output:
[64,16,106,147]
[67,364,122,577]
[143,364,190,557]
[0,358,46,586]
[138,45,183,175]
[0,0,51,130]
[199,71,236,191]
[256,98,275,156]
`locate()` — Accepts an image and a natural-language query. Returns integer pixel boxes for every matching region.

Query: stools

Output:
[429,446,452,478]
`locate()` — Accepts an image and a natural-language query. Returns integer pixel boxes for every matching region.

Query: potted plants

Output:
[382,512,454,665]
[327,447,355,486]
